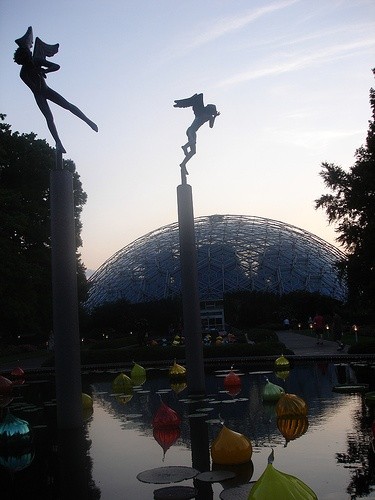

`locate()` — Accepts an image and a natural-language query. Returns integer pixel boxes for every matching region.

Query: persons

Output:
[12,27,99,153]
[172,92,220,176]
[151,327,184,359]
[331,311,345,348]
[136,315,150,347]
[313,311,325,343]
[48,329,56,354]
[202,327,249,344]
